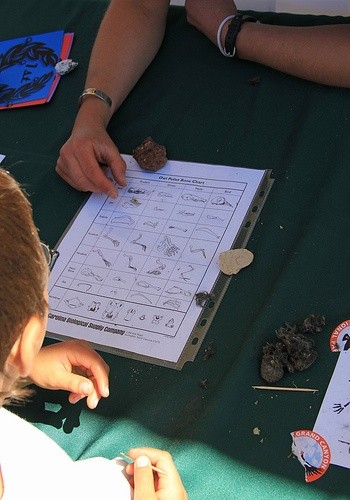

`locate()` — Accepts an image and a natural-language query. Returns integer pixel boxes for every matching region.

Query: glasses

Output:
[40,242,60,278]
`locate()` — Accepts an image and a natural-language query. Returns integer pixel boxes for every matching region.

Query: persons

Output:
[55,0,350,199]
[0,167,189,500]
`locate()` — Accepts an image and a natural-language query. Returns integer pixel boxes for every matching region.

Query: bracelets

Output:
[77,87,113,110]
[225,13,257,57]
[216,14,236,57]
